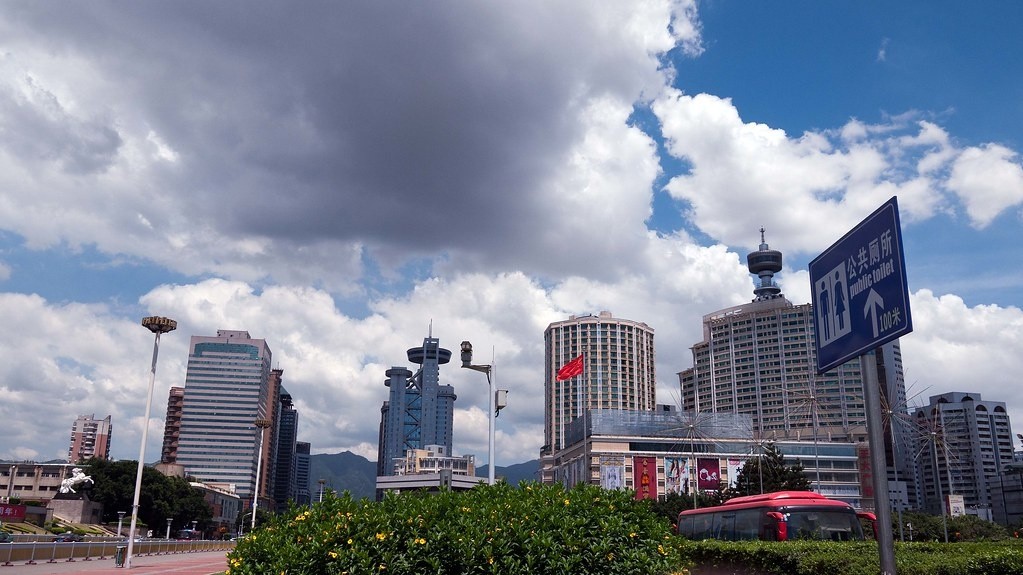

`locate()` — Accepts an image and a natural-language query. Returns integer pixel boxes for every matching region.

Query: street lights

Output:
[125,316,177,568]
[998,472,1011,536]
[250,419,272,534]
[461,340,495,486]
[166,519,173,539]
[317,478,326,503]
[192,521,197,530]
[241,513,252,535]
[117,512,125,538]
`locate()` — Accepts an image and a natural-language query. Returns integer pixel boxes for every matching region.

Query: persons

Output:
[669,459,687,492]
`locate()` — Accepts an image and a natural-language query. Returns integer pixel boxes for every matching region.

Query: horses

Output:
[59,468,94,493]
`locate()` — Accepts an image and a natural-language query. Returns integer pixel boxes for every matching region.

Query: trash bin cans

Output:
[116,546,127,568]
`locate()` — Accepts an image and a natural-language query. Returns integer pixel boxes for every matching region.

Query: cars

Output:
[0,532,14,543]
[51,532,83,542]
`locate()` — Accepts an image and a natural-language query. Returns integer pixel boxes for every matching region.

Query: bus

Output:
[176,529,201,542]
[678,490,878,541]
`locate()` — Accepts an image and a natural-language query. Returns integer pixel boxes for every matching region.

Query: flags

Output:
[557,354,585,381]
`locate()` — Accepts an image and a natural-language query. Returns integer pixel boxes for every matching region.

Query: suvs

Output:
[223,533,244,542]
